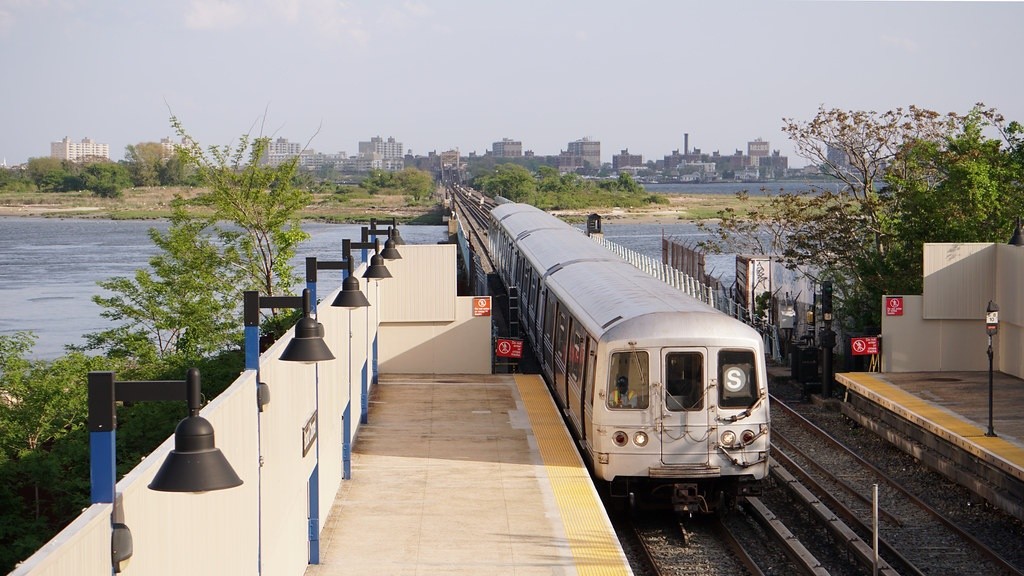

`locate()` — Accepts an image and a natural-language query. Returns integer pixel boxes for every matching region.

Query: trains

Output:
[487,202,772,517]
